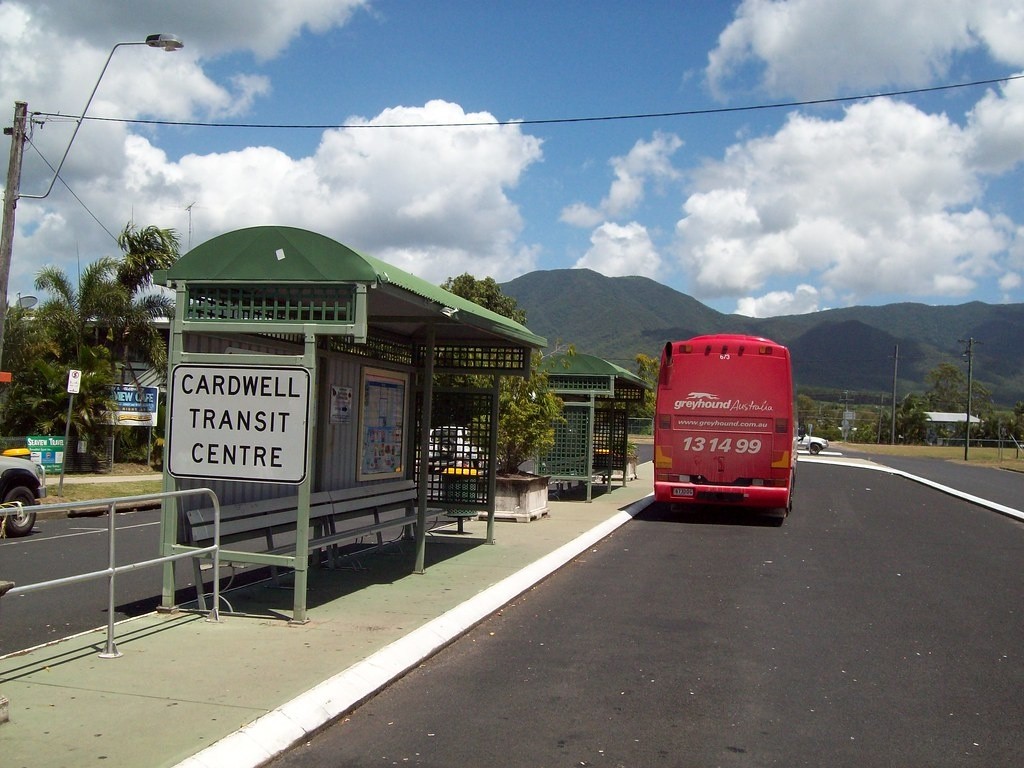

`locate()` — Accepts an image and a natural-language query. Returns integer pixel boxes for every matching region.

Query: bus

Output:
[654,334,798,527]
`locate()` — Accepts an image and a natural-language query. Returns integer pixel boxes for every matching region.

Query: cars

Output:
[798,433,829,454]
[0,456,47,537]
[428,426,483,471]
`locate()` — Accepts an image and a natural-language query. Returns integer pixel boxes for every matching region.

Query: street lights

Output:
[0,34,184,371]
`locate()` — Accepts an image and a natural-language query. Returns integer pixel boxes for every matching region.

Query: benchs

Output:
[186,480,449,618]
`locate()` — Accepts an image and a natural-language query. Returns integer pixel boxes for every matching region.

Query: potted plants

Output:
[466,370,568,523]
[602,436,639,481]
